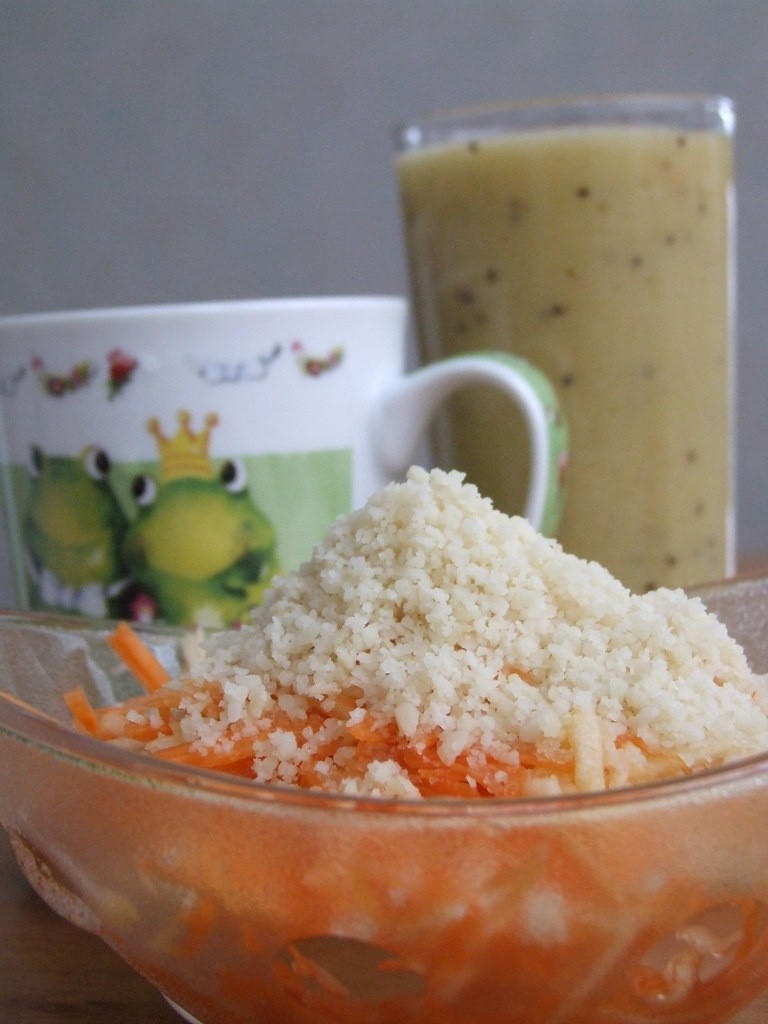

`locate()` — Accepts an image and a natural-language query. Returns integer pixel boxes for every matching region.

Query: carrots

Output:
[0,622,768,1024]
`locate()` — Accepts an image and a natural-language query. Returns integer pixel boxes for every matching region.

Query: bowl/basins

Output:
[0,576,768,1024]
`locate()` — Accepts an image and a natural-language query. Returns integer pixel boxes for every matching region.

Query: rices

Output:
[122,462,768,802]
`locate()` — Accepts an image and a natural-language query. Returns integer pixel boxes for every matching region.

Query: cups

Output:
[1,295,567,630]
[393,93,737,592]
